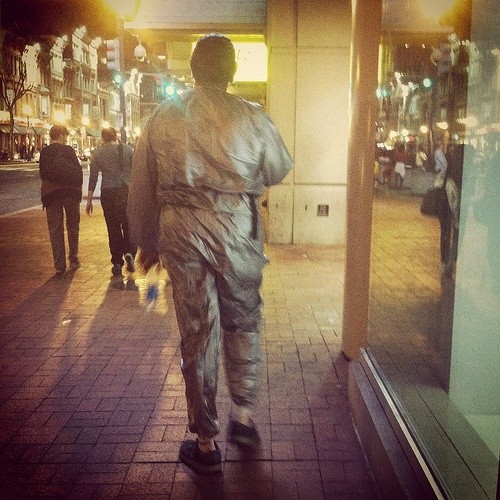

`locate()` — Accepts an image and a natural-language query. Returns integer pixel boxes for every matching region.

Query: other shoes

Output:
[178,440,222,474]
[226,414,261,450]
[124,252,135,272]
[56,269,64,274]
[112,266,122,276]
[70,261,81,268]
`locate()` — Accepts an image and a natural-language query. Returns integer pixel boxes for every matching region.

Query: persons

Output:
[126,34,294,476]
[85,124,138,275]
[388,138,477,281]
[39,124,83,272]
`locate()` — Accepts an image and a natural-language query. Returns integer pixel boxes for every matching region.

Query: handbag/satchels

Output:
[116,181,129,210]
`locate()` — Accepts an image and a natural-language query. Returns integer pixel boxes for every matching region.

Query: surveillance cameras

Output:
[134,45,146,61]
